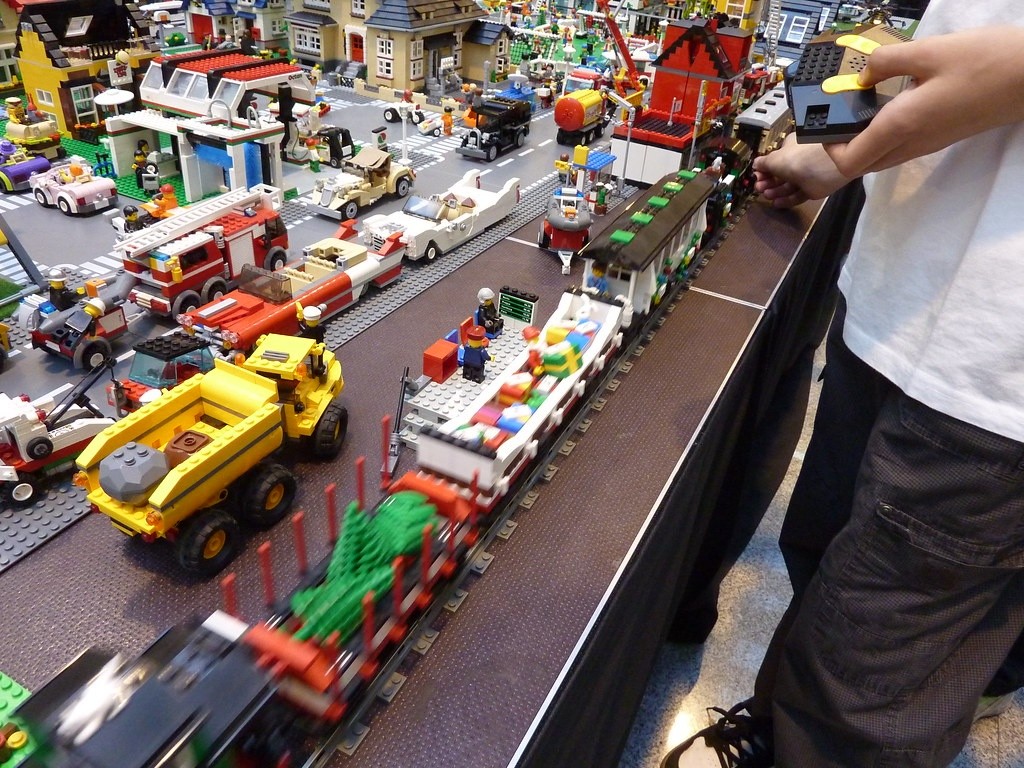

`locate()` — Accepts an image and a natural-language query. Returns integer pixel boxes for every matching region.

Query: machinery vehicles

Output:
[73,331,349,579]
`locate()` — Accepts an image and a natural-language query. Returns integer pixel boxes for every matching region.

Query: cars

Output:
[361,169,520,267]
[27,155,120,216]
[174,218,407,360]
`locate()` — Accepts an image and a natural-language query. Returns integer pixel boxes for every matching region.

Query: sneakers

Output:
[660,702,774,768]
[973,691,1014,722]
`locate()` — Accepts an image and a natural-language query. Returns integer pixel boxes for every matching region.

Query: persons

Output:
[132,139,151,189]
[7,96,30,126]
[603,34,612,52]
[562,27,569,47]
[202,29,232,50]
[523,325,544,378]
[582,260,610,298]
[542,2,547,8]
[572,8,576,18]
[544,63,554,78]
[298,306,327,344]
[441,106,454,135]
[462,82,485,106]
[401,89,413,103]
[48,268,77,311]
[0,140,17,164]
[564,201,577,221]
[54,297,106,350]
[505,1,512,14]
[521,3,533,24]
[113,49,130,78]
[59,164,84,184]
[550,18,559,35]
[123,184,178,233]
[655,0,1023,767]
[532,34,540,53]
[558,153,571,181]
[478,288,501,335]
[241,30,256,56]
[250,102,259,119]
[463,325,495,383]
[511,16,518,27]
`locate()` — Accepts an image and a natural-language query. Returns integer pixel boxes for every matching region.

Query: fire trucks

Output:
[27,179,289,374]
